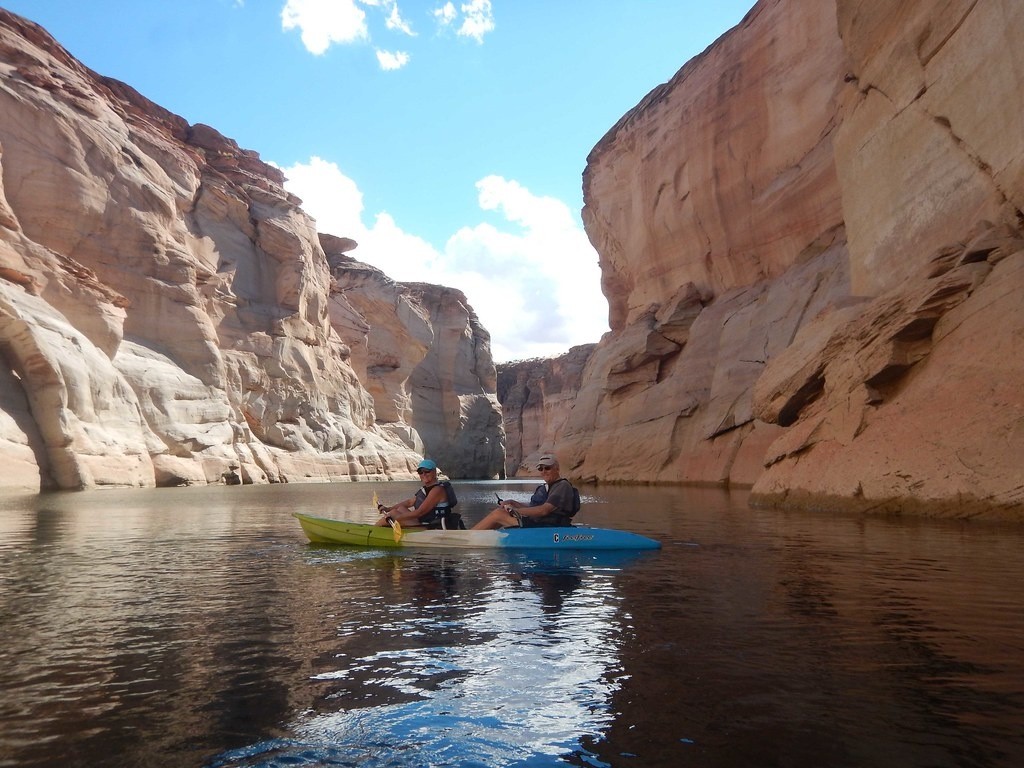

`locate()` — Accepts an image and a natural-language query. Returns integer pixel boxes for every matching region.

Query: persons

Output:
[470,453,581,530]
[375,460,457,528]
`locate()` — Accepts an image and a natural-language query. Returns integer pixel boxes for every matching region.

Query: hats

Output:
[536,453,559,467]
[417,460,436,472]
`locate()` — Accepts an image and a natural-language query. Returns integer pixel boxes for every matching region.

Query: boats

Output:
[292,512,663,552]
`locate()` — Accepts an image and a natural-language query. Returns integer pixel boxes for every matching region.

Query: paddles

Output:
[493,491,520,517]
[372,489,402,543]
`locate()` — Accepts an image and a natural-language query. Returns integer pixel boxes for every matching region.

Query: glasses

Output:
[418,470,431,474]
[538,466,551,471]
